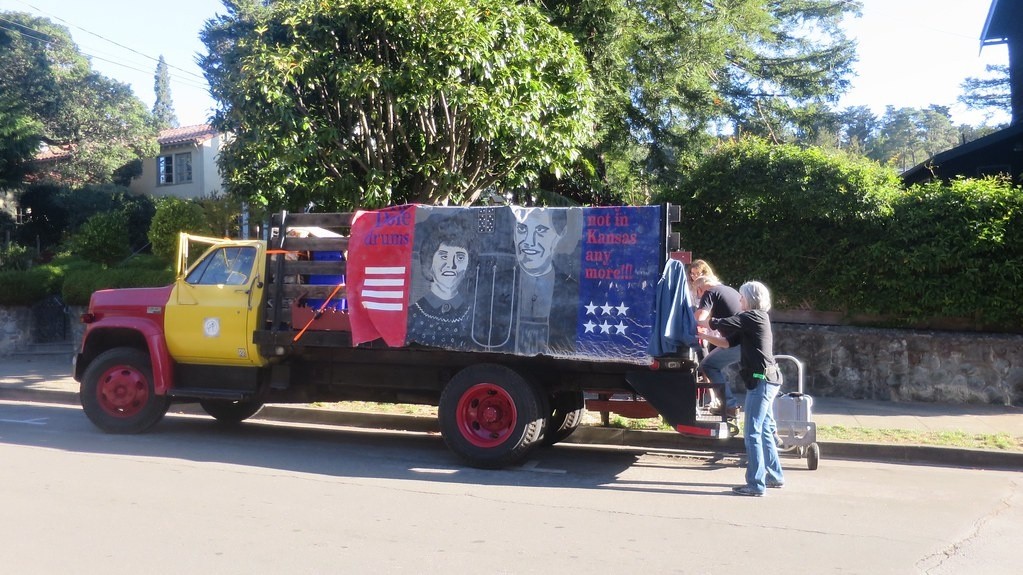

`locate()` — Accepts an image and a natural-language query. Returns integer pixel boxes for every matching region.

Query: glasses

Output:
[689,272,702,277]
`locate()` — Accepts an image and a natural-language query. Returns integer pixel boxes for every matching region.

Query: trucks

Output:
[73,202,740,470]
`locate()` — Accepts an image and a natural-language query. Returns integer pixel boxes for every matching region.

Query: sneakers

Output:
[766,482,783,488]
[732,484,763,497]
[710,405,741,418]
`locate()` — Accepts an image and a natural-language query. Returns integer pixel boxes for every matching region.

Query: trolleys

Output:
[767,355,819,471]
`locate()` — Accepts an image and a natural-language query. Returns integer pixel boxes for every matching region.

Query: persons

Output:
[688,260,782,447]
[696,282,783,497]
